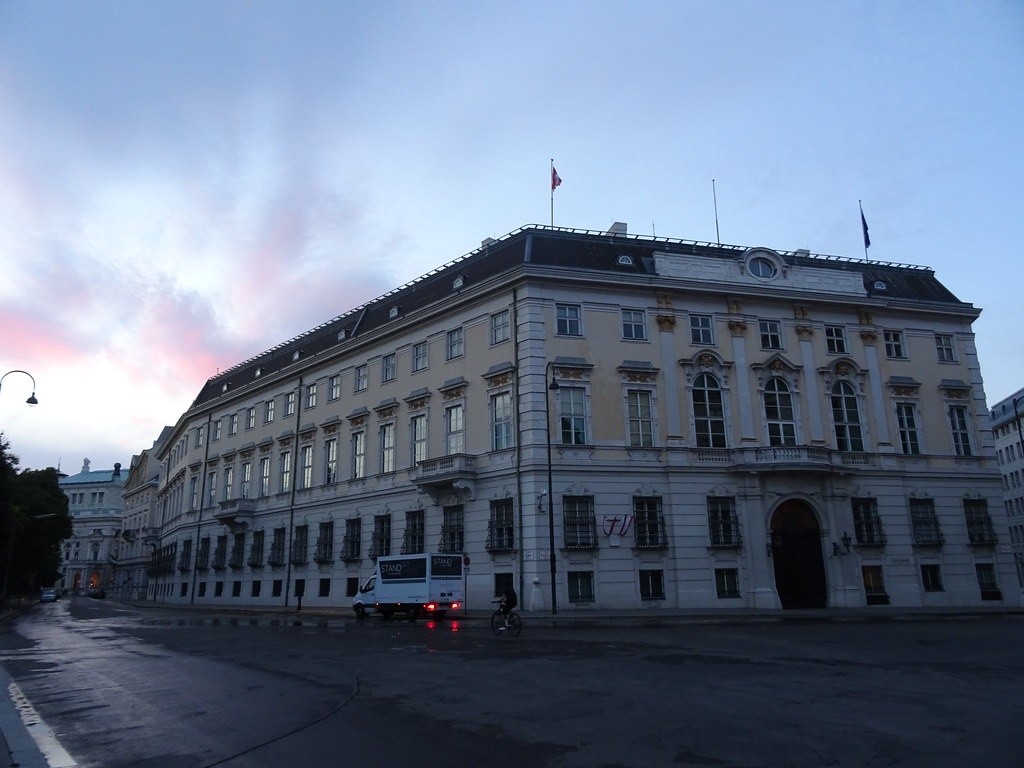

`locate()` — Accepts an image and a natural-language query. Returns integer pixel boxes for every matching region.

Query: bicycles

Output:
[490,599,522,638]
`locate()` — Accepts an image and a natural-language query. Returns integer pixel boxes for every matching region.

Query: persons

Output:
[497,580,518,631]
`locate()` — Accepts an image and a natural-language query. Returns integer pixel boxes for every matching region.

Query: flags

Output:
[859,199,871,248]
[552,166,562,192]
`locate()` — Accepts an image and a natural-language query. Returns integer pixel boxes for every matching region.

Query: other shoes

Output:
[510,614,517,621]
[498,627,506,631]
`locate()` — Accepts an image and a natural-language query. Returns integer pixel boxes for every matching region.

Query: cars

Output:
[91,590,106,600]
[41,589,59,602]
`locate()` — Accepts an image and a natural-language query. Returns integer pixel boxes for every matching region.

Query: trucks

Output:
[353,552,467,619]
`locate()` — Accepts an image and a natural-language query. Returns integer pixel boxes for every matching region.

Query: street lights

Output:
[542,361,558,614]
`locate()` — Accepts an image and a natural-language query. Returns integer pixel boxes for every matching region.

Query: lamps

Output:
[766,531,783,557]
[832,531,855,557]
